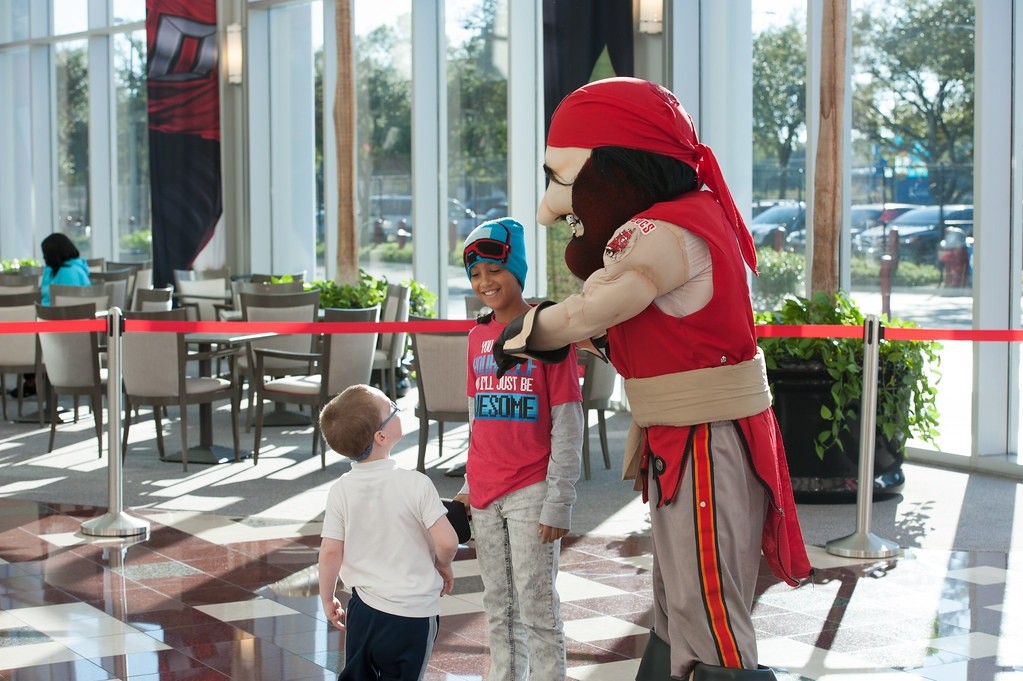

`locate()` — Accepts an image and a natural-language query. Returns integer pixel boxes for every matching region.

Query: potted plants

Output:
[747,288,948,497]
[304,273,436,397]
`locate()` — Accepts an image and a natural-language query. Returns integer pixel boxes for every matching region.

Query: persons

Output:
[9,234,93,398]
[320,384,459,681]
[453,217,586,681]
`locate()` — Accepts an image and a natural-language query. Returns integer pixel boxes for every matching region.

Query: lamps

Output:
[639,0,664,35]
[226,23,243,84]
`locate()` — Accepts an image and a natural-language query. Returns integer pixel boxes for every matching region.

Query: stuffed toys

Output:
[491,76,814,681]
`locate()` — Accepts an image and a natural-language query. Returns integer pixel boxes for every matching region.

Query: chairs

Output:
[0,257,549,478]
[577,349,616,481]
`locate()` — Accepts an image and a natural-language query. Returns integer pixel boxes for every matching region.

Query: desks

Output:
[159,329,291,463]
[217,308,325,427]
[10,310,109,424]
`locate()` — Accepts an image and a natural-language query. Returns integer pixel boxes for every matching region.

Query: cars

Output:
[784,202,926,255]
[749,201,807,247]
[851,203,975,259]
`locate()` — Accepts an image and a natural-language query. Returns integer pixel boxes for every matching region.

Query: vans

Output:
[366,192,474,240]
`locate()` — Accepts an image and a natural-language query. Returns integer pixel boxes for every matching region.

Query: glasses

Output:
[374,400,401,434]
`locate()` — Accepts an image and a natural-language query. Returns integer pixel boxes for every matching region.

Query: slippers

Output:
[10,383,37,398]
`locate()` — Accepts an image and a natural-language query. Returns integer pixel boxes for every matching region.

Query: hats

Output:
[463,216,528,293]
